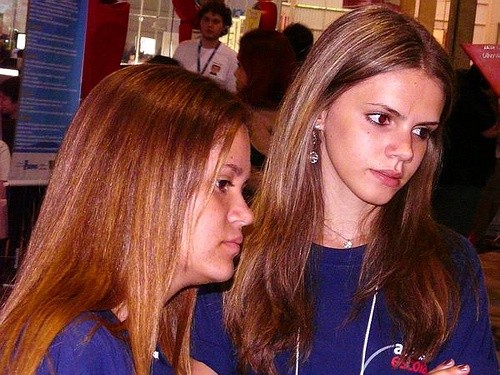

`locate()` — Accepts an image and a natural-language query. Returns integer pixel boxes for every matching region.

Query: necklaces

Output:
[324,223,368,249]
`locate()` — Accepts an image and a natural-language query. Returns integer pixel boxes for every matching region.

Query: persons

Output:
[0,64,254,375]
[189,10,500,375]
[149,3,313,204]
[428,65,500,249]
